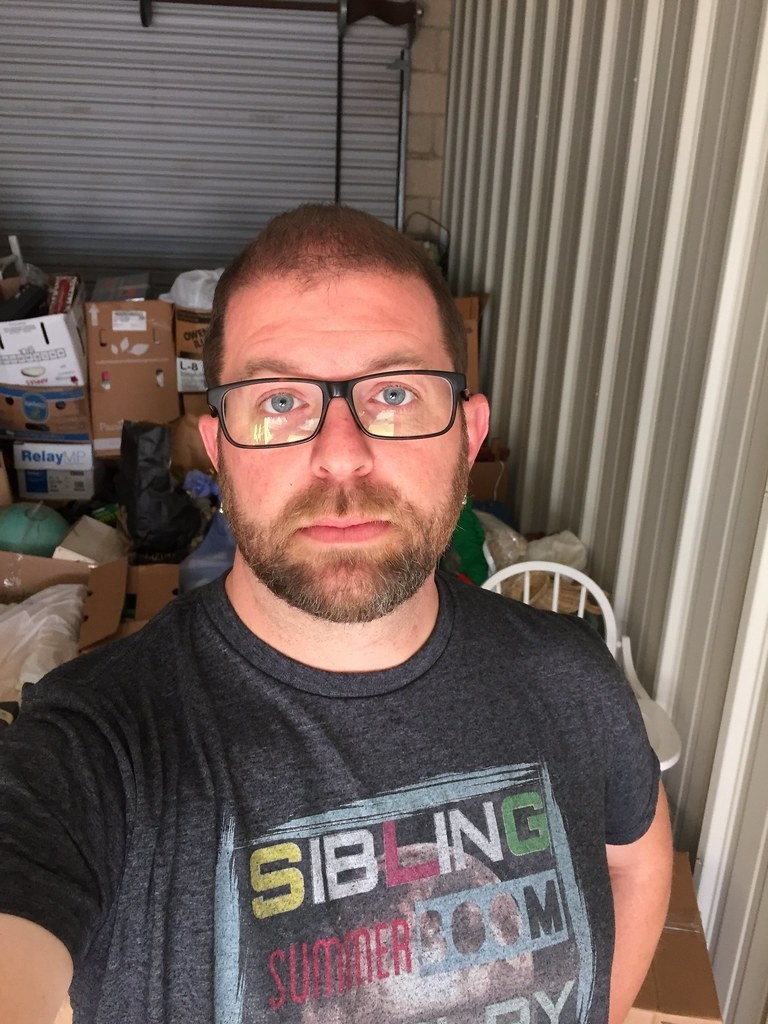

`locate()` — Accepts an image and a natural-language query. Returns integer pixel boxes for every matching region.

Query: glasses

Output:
[206,370,468,449]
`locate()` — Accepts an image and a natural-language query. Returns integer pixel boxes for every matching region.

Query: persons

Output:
[0,205,675,1024]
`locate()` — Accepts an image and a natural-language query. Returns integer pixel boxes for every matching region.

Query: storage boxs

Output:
[0,272,611,729]
[622,849,724,1024]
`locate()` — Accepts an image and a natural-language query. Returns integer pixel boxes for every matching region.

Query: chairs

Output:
[480,561,682,771]
[0,235,24,281]
